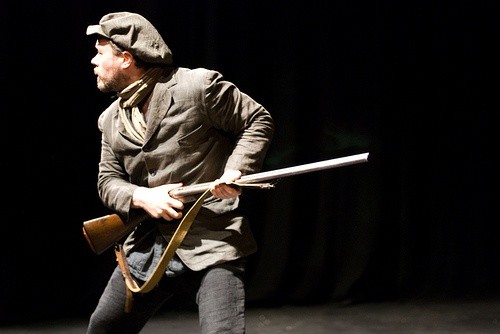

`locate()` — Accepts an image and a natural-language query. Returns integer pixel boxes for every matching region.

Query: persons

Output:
[87,12,276,334]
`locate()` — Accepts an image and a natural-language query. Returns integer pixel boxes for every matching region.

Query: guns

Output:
[80,152,371,256]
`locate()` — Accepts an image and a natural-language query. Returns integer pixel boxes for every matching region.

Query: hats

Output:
[86,11,172,65]
